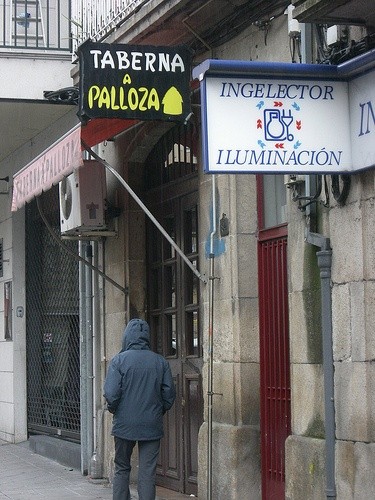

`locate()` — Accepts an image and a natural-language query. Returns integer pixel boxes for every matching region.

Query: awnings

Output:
[8,117,209,287]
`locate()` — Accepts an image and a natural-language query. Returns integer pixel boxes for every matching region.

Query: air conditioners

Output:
[59,157,114,235]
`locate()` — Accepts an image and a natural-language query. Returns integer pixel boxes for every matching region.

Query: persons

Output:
[102,318,176,499]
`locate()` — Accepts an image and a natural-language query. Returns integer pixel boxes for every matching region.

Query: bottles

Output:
[89,452,101,478]
